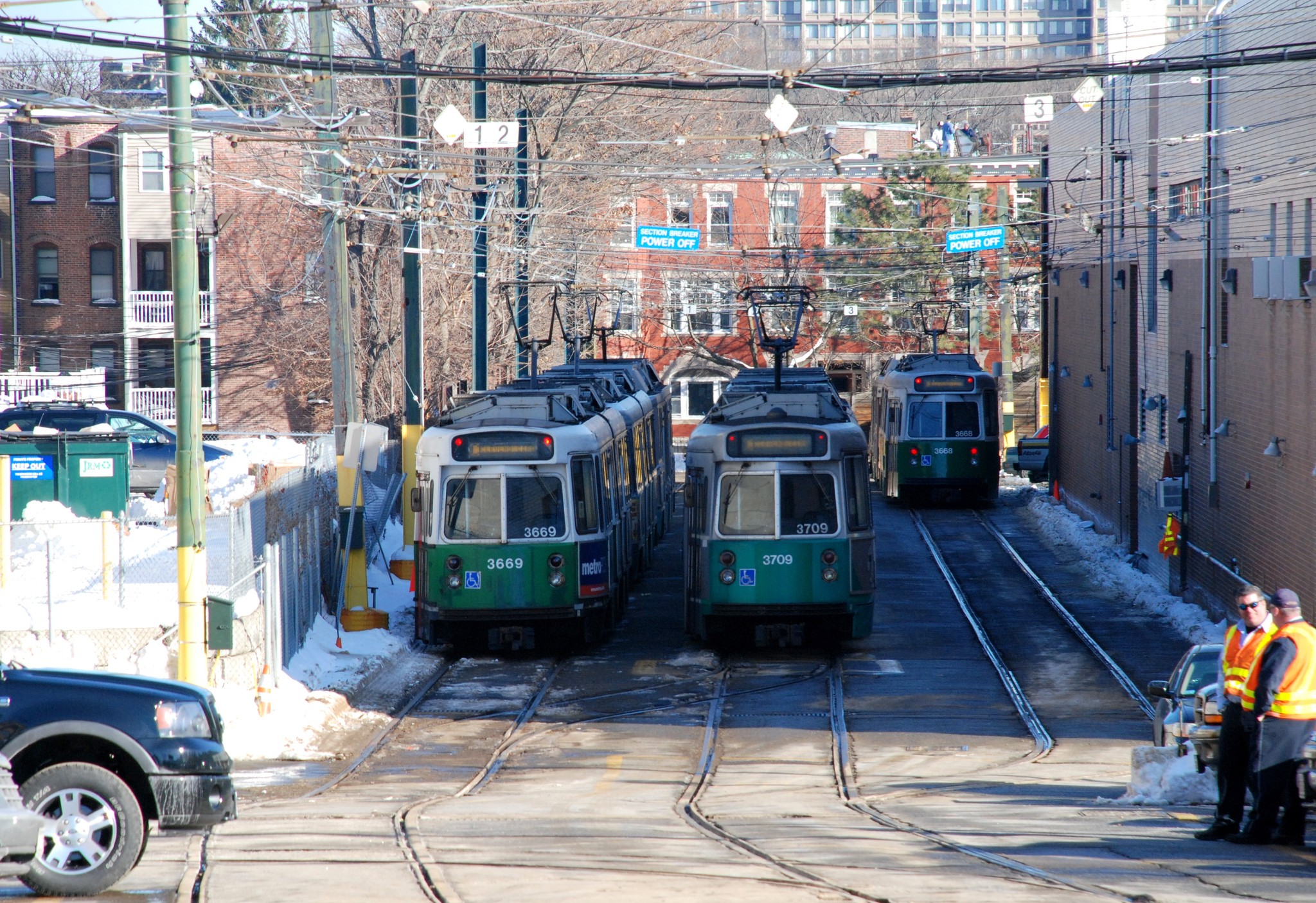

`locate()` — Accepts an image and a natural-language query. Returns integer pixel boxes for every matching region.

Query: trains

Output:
[407,358,673,634]
[681,361,868,642]
[871,351,998,508]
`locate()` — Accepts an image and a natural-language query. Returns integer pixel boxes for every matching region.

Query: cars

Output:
[0,661,236,893]
[1148,639,1223,767]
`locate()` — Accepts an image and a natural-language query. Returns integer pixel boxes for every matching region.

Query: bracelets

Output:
[1220,708,1227,713]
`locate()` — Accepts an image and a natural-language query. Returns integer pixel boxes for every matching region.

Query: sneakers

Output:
[1194,818,1240,841]
[1228,826,1270,845]
[1266,829,1306,848]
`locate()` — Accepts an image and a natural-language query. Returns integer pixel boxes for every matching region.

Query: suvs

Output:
[7,398,228,497]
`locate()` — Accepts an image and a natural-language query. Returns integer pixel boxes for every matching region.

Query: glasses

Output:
[1235,598,1265,610]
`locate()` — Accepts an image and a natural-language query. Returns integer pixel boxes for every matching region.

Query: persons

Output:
[1195,584,1279,842]
[1231,589,1316,845]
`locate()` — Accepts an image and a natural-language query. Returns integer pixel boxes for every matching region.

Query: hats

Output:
[1269,587,1302,609]
